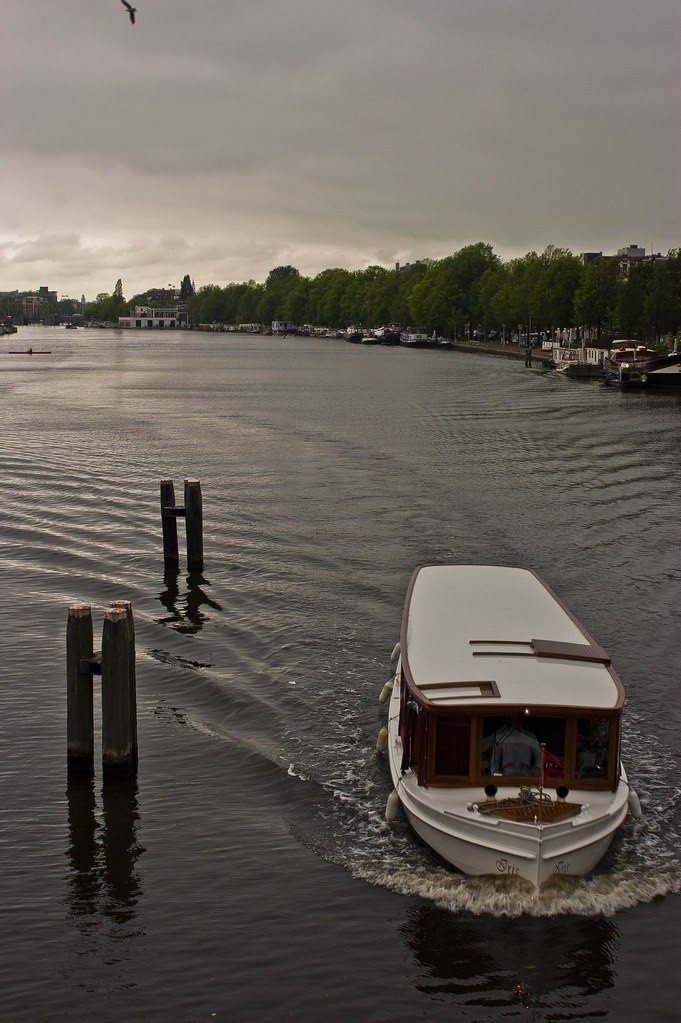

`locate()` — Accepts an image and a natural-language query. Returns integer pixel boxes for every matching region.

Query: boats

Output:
[386,564,642,897]
[551,329,658,386]
[400,325,434,347]
[361,334,377,344]
[434,335,451,347]
[379,330,400,345]
[9,351,51,354]
[343,323,367,344]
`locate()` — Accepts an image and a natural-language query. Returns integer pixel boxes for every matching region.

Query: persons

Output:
[491,718,608,778]
